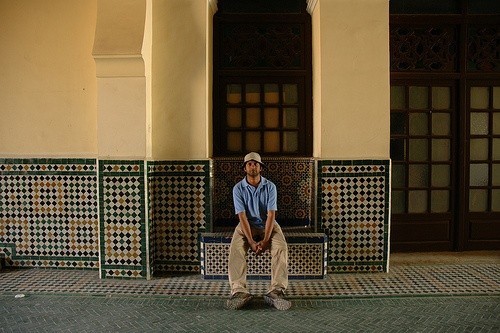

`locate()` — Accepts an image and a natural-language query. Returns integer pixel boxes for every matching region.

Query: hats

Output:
[240,151,268,171]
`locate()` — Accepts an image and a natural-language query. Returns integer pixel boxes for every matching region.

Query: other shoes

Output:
[265,289,291,311]
[227,291,253,309]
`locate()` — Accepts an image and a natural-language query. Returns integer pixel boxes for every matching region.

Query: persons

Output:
[225,152,293,311]
